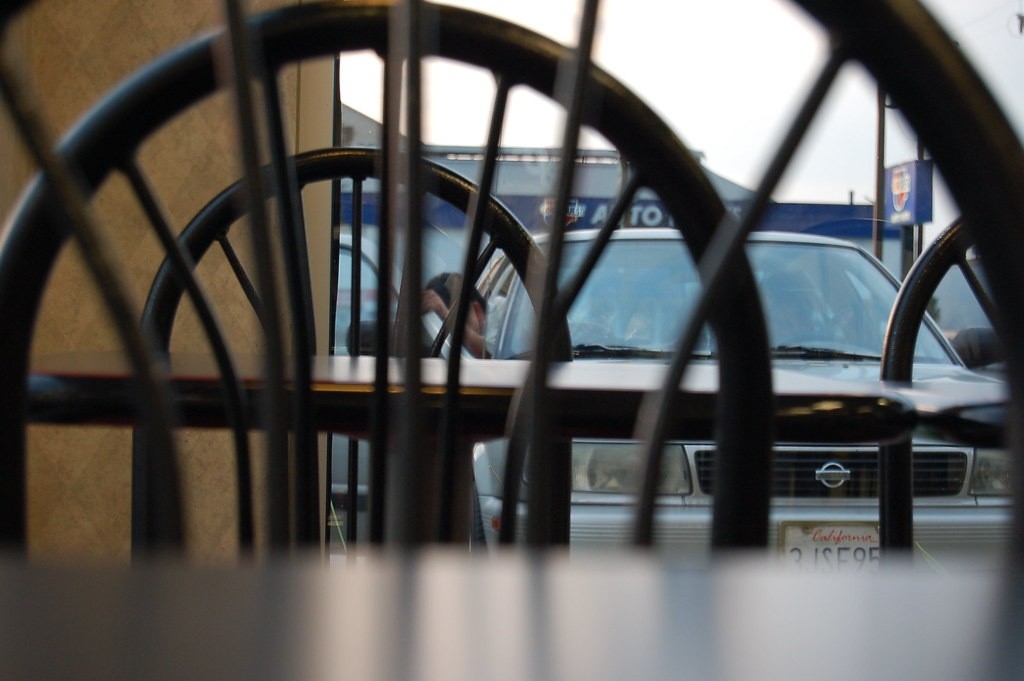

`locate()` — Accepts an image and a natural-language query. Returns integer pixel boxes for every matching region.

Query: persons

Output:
[421,274,507,359]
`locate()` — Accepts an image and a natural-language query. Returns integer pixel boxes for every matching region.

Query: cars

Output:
[334,227,1015,558]
